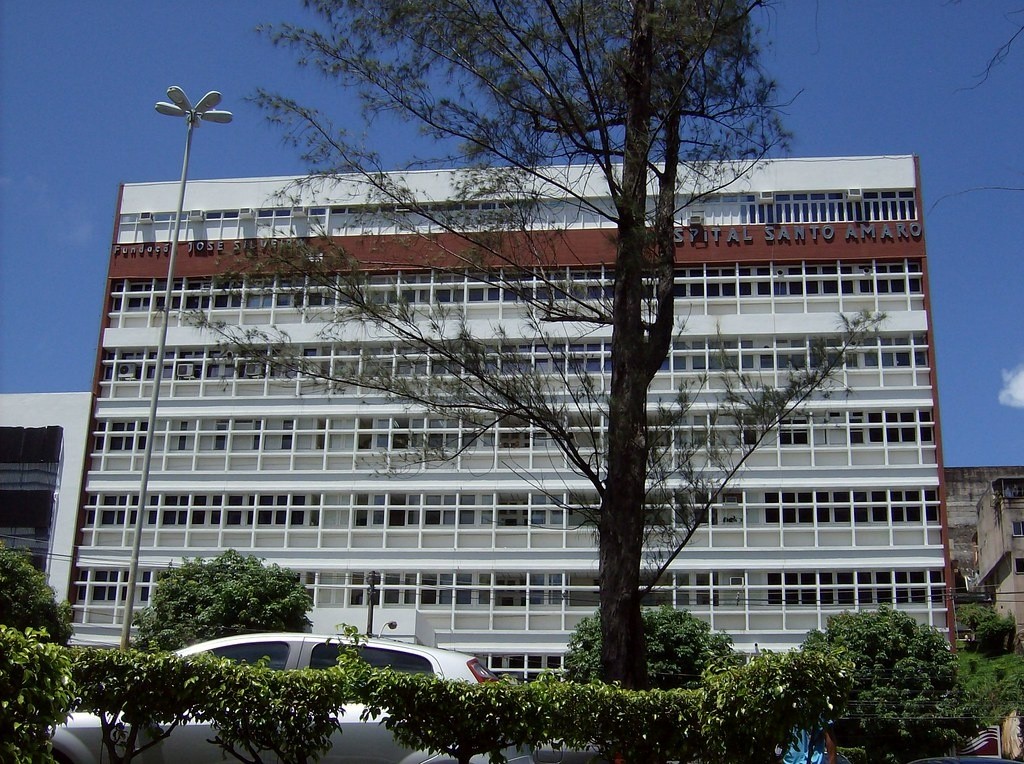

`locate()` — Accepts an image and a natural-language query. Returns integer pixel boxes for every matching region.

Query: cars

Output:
[39,633,536,764]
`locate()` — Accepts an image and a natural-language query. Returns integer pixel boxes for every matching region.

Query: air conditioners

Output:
[176,363,195,376]
[689,216,702,225]
[245,363,263,376]
[190,209,204,222]
[395,204,412,212]
[118,363,137,377]
[239,208,254,218]
[139,212,154,224]
[292,206,307,217]
[730,577,745,586]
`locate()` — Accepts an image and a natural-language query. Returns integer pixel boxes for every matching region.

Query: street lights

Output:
[118,84,232,650]
